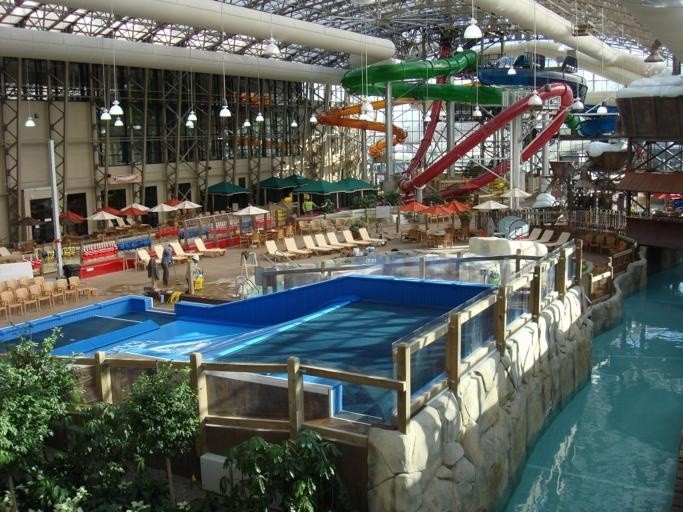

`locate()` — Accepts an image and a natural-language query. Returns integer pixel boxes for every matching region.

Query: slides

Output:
[479,47,621,142]
[342,35,585,131]
[398,35,574,200]
[316,96,414,175]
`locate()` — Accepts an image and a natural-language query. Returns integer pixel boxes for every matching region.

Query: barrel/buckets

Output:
[498,216,529,239]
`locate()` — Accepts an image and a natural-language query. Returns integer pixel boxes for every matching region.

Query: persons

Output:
[146,254,160,288]
[160,245,175,288]
[184,253,202,294]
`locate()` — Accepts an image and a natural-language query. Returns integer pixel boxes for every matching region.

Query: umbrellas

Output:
[395,186,533,234]
[8,198,203,241]
[230,206,271,217]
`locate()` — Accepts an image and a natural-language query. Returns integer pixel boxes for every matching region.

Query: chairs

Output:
[0,217,226,321]
[409,227,464,248]
[523,227,628,255]
[240,219,387,261]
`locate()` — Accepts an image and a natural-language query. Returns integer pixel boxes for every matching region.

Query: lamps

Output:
[24,3,433,131]
[529,0,542,107]
[472,43,483,119]
[464,0,483,38]
[572,1,583,110]
[645,40,664,62]
[507,28,516,75]
[597,5,608,116]
